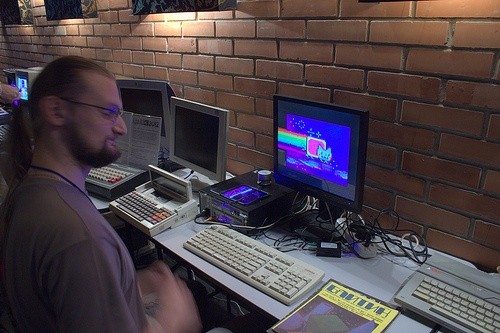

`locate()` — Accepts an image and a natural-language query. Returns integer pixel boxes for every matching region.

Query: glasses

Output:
[62,98,122,122]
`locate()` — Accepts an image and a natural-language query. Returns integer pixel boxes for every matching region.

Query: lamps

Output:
[0,0,34,25]
[44,0,99,22]
[131,0,238,16]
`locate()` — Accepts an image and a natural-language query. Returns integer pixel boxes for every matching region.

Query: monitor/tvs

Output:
[15,68,43,101]
[116,78,175,143]
[273,95,370,245]
[169,95,229,191]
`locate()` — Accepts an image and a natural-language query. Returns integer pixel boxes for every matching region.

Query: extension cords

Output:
[335,217,377,259]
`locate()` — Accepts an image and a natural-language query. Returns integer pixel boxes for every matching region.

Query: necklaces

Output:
[30,165,96,207]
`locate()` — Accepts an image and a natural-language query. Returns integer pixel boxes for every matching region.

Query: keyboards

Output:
[89,166,130,183]
[393,264,500,333]
[182,225,325,305]
[115,192,174,225]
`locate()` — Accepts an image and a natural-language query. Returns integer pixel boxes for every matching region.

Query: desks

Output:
[134,167,486,333]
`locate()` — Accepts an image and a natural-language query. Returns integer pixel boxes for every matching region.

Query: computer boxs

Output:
[199,168,308,236]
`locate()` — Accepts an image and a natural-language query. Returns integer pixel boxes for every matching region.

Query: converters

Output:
[316,241,341,258]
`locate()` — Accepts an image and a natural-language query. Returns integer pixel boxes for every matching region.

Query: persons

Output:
[0,56,274,333]
